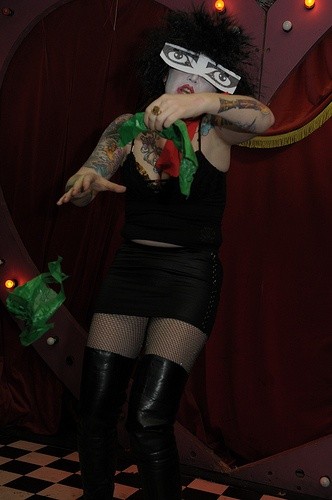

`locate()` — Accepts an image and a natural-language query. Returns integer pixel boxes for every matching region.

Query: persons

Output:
[56,9,277,500]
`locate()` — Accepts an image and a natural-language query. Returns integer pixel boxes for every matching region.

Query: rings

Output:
[65,185,73,191]
[152,105,163,116]
